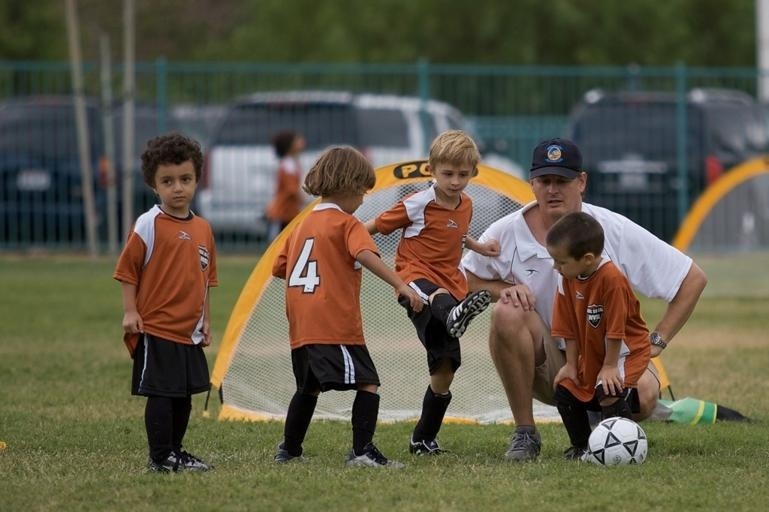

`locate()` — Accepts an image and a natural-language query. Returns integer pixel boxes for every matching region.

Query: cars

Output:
[0,95,133,246]
[82,101,225,249]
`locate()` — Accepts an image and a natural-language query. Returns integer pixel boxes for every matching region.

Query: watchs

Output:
[650,330,668,350]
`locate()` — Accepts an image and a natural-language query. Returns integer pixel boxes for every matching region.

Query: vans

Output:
[552,80,768,256]
[199,81,530,259]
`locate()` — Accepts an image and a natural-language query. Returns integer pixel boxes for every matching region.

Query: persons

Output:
[460,138,708,462]
[114,134,218,470]
[546,211,650,459]
[365,131,502,456]
[263,126,305,230]
[271,147,421,468]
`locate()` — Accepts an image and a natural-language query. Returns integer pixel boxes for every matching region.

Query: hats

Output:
[529,138,583,180]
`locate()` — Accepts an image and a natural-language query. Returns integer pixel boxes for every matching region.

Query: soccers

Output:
[588,418,647,466]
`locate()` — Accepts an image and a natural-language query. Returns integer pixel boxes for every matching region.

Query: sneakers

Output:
[409,433,444,458]
[505,429,540,461]
[346,441,405,472]
[445,290,491,338]
[274,443,305,465]
[148,450,208,475]
